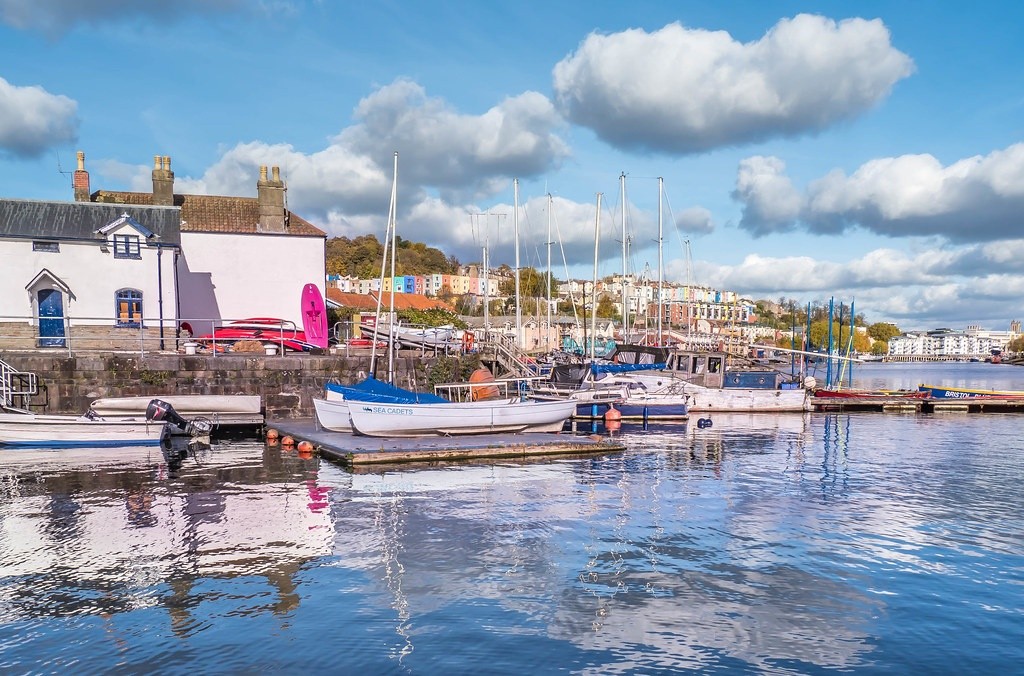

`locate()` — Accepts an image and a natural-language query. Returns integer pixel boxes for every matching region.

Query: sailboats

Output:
[513,171,812,420]
[325,151,521,404]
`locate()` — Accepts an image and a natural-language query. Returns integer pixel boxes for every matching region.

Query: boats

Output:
[312,397,536,433]
[0,399,213,446]
[345,397,579,438]
[918,384,1024,399]
[814,388,932,399]
[344,324,477,351]
[359,324,453,352]
[201,318,306,352]
